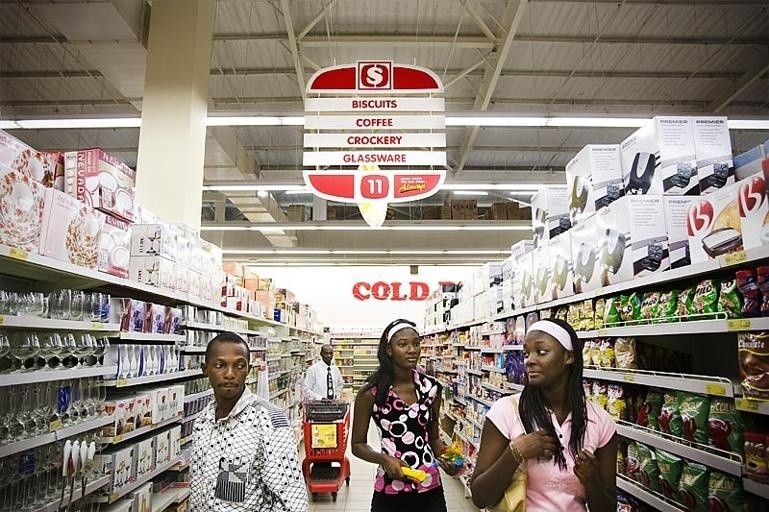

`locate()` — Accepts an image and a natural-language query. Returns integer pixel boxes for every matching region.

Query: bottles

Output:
[266,341,279,391]
[444,422,464,477]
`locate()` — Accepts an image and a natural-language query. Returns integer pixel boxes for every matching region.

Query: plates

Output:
[99,170,133,212]
[64,207,130,268]
[0,150,66,256]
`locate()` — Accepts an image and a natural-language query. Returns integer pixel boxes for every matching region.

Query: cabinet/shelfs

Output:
[418,244,767,511]
[1,244,326,512]
[322,332,384,394]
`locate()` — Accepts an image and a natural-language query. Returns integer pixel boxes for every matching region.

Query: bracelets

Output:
[509,440,525,465]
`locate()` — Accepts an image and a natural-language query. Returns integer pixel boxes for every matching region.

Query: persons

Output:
[351,319,467,512]
[302,344,343,403]
[470,318,619,511]
[187,333,311,512]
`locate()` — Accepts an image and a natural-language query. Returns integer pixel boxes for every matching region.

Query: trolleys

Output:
[298,400,351,505]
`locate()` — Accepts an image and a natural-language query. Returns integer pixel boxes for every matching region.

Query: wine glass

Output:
[0,332,112,374]
[145,227,161,253]
[1,378,108,446]
[142,258,160,287]
[0,428,103,512]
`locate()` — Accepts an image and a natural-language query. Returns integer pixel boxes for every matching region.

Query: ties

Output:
[327,367,334,399]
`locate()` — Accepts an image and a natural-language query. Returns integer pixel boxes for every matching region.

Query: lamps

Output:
[203,181,567,192]
[0,110,769,129]
[201,220,532,231]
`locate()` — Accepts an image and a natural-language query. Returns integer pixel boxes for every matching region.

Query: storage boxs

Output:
[1,132,322,331]
[409,116,769,327]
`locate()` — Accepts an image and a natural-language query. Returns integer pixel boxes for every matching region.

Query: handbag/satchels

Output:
[486,470,527,512]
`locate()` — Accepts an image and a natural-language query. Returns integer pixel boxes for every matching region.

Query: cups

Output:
[180,304,225,326]
[180,354,207,373]
[0,288,110,323]
[179,328,217,349]
